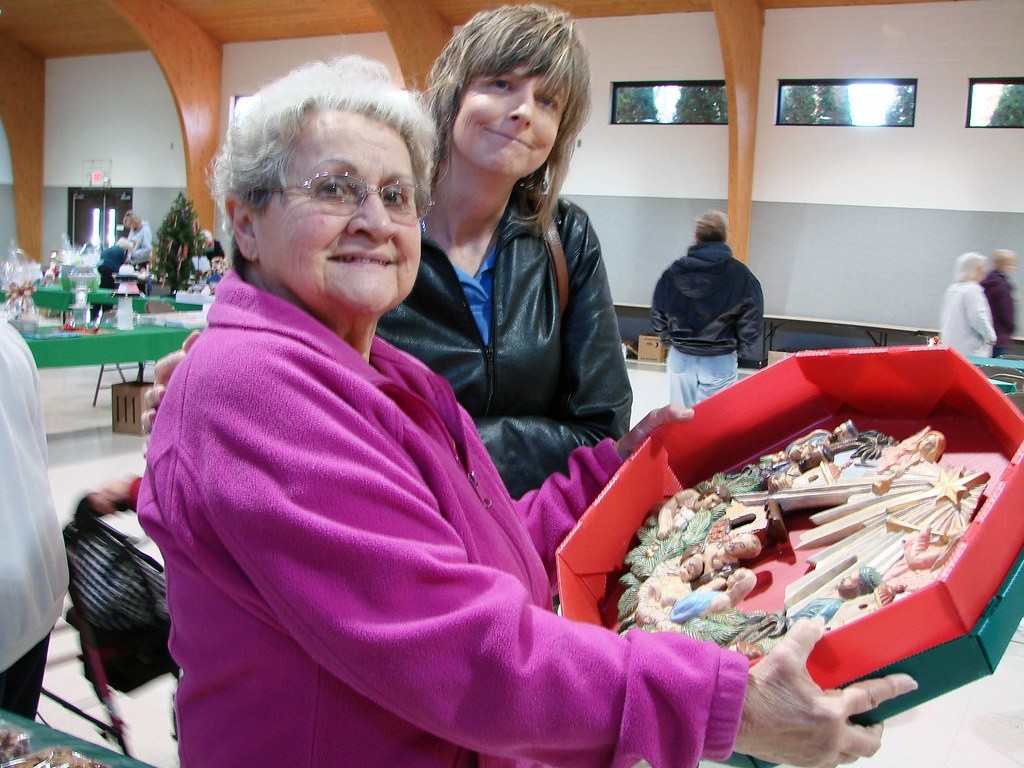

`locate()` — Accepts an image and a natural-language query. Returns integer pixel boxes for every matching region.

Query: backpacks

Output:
[61,513,179,694]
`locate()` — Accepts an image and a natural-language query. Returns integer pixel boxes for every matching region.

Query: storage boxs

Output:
[637,334,667,364]
[112,381,157,436]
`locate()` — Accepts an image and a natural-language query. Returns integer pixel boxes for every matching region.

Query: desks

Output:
[960,354,1024,378]
[0,283,122,311]
[131,294,206,321]
[12,310,207,368]
[0,703,161,768]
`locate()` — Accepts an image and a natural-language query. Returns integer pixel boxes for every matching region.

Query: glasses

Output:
[253,172,436,222]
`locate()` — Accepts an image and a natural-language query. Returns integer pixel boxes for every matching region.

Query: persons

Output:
[133,50,922,767]
[936,252,997,359]
[86,208,226,323]
[978,248,1017,360]
[0,314,69,723]
[141,0,634,499]
[648,210,764,410]
